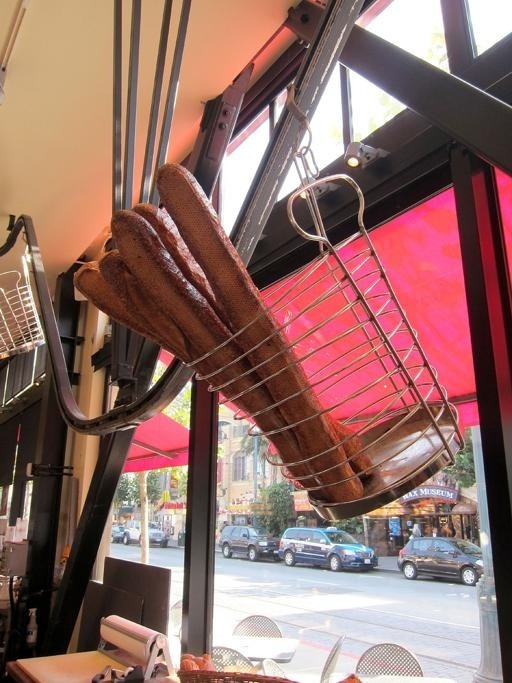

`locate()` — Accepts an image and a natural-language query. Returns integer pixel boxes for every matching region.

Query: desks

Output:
[5,645,135,682]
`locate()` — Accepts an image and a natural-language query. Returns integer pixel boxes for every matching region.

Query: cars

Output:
[111,525,124,543]
[397,535,484,586]
[278,527,379,572]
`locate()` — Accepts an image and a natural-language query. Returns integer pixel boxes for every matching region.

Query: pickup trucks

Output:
[123,519,170,548]
[218,524,280,561]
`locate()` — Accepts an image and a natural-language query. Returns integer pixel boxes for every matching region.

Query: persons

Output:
[428,527,443,538]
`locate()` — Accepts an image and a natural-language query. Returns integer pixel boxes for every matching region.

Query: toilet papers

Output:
[101,615,165,662]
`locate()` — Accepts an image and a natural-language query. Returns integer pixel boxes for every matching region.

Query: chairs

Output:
[231,612,426,683]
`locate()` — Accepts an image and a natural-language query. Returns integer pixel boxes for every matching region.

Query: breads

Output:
[178,654,214,671]
[72,162,374,501]
